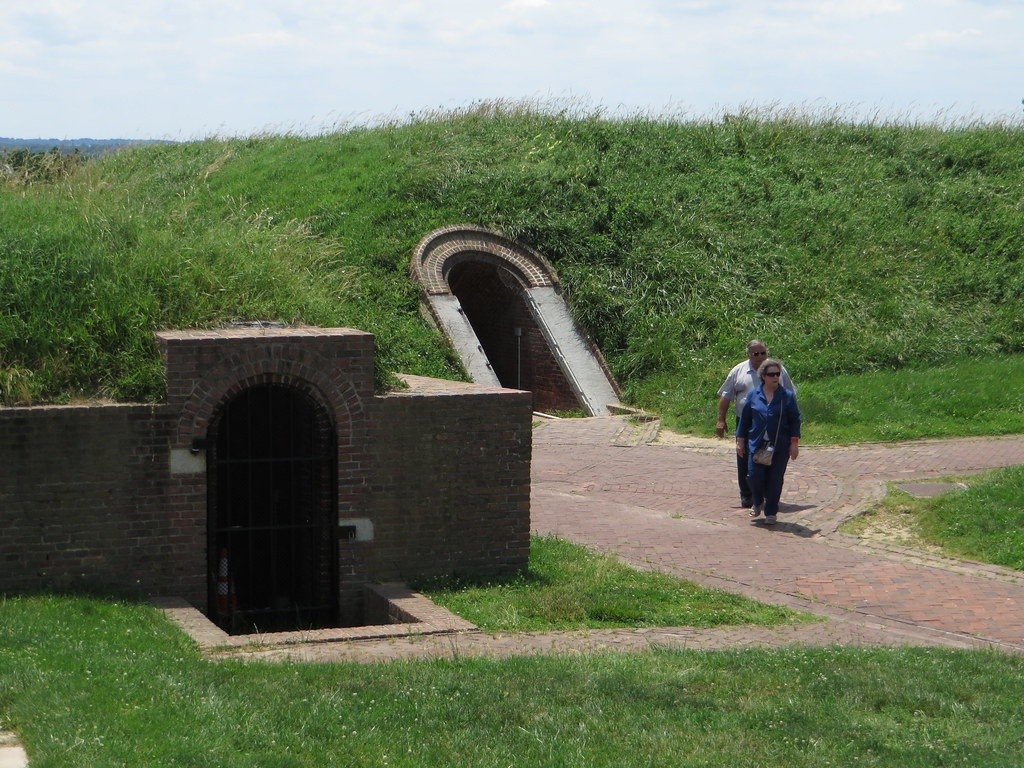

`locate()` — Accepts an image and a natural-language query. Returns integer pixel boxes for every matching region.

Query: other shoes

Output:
[741,495,753,508]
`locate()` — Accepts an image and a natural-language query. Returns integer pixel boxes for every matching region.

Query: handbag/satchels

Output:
[753,447,774,466]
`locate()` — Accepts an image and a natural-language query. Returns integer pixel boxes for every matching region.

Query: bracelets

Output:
[790,438,798,443]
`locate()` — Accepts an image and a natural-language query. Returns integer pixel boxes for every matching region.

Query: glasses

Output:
[763,372,780,377]
[748,352,766,357]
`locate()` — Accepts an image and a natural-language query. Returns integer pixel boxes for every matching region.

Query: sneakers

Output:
[765,515,777,525]
[750,503,764,518]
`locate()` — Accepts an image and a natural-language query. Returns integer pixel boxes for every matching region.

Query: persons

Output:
[717,339,795,507]
[738,357,801,525]
